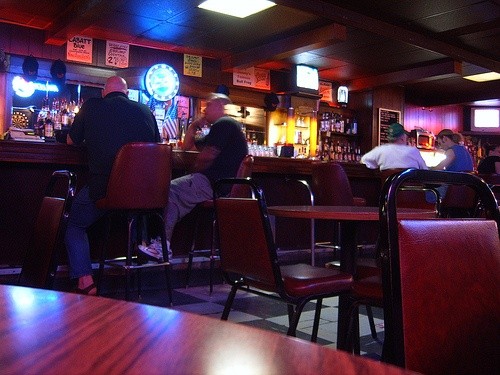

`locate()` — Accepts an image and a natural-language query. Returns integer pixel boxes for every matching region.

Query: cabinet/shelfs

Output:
[318,112,363,159]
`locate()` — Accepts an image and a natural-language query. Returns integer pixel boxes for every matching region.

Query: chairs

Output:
[379,162,500,375]
[15,169,79,288]
[209,173,354,344]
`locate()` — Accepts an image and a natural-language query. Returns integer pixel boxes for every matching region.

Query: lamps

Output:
[337,85,349,104]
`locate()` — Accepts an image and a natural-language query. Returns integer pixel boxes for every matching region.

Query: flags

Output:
[163,97,179,138]
[145,95,156,116]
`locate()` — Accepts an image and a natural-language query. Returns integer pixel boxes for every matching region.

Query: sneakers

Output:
[138,238,172,261]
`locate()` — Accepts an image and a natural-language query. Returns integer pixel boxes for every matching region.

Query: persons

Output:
[425,129,474,203]
[137,93,247,262]
[360,122,428,172]
[55,75,161,295]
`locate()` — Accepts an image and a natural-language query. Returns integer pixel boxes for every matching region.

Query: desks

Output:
[266,204,443,357]
[0,283,426,375]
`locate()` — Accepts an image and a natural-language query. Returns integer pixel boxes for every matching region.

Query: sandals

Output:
[74,283,98,296]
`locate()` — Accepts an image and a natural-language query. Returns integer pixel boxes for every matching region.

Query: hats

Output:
[388,123,410,137]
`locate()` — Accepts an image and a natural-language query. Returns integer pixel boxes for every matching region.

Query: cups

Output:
[247,145,277,157]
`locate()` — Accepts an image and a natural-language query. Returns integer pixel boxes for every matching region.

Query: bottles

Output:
[245,127,263,145]
[177,113,214,150]
[33,97,84,141]
[315,112,362,161]
[296,116,307,158]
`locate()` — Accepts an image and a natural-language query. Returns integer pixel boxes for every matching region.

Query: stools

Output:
[313,161,370,269]
[94,141,179,304]
[186,153,259,294]
